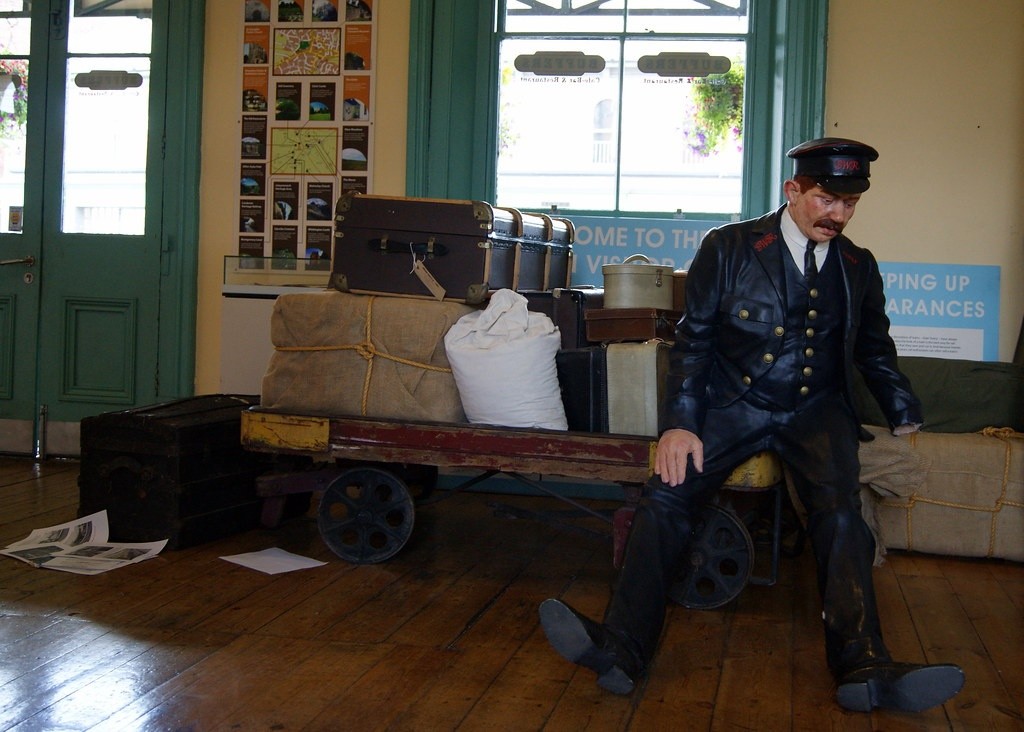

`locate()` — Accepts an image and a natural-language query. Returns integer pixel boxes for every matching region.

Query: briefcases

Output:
[330,191,576,303]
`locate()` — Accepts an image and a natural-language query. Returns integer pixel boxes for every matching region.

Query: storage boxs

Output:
[74,386,260,548]
[332,194,687,442]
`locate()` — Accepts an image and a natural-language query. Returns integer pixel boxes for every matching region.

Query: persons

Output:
[537,137,965,713]
